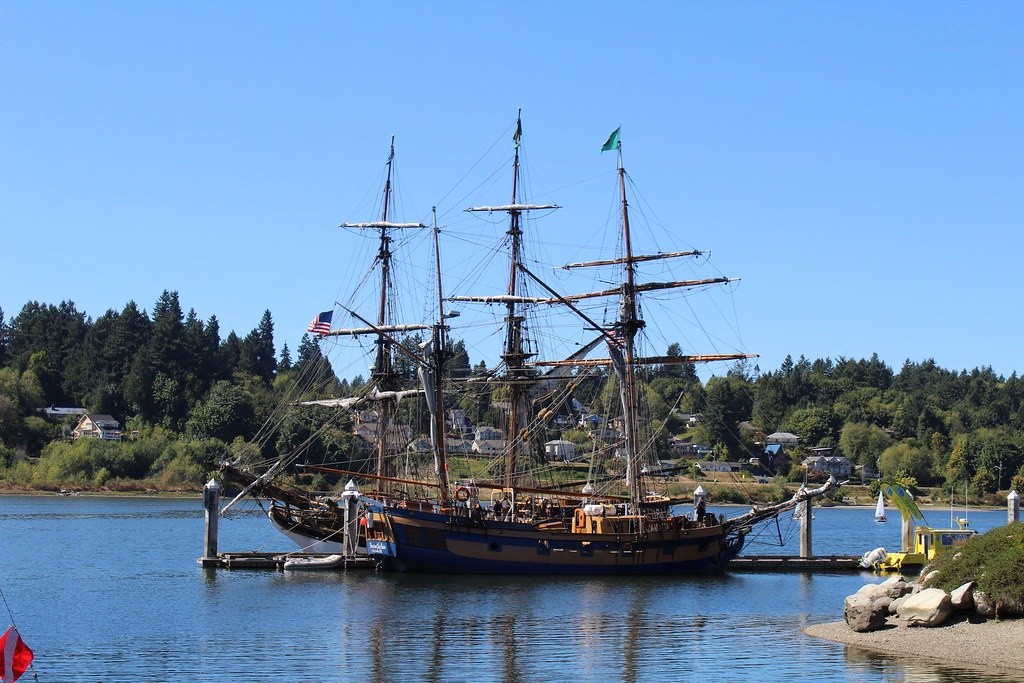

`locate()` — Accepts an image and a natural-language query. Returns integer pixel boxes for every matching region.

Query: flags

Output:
[512,124,522,144]
[606,330,625,350]
[600,127,620,154]
[307,310,334,336]
[385,145,394,164]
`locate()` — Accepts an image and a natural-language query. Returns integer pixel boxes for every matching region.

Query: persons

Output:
[694,496,705,529]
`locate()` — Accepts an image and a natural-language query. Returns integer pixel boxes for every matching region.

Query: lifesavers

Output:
[454,486,471,503]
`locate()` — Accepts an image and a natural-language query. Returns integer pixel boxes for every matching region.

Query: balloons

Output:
[360,517,366,526]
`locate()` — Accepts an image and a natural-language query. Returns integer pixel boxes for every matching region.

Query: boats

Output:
[196,106,843,575]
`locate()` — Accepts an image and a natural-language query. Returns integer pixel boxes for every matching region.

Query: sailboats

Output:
[792,483,816,520]
[872,487,890,523]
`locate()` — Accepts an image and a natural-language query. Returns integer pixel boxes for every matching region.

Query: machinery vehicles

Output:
[857,482,979,575]
